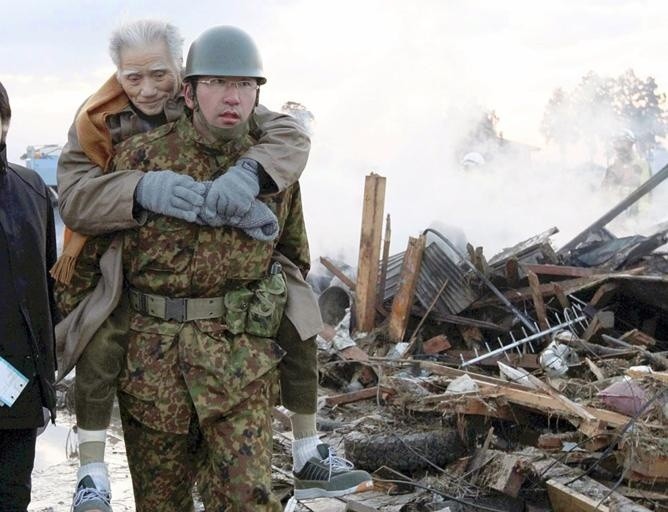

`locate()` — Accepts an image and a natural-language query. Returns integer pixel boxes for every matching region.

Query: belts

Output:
[128,286,226,325]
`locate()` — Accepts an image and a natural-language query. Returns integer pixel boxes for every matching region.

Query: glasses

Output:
[195,78,259,92]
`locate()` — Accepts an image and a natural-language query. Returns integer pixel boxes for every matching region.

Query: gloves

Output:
[206,158,269,225]
[135,169,206,223]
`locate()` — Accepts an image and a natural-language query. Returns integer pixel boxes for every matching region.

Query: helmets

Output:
[181,24,267,86]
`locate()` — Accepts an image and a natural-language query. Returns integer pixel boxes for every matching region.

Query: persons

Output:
[0,81,57,512]
[54,26,311,511]
[54,20,373,511]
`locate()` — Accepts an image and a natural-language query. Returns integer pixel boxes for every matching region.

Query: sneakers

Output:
[72,475,113,512]
[292,443,373,500]
